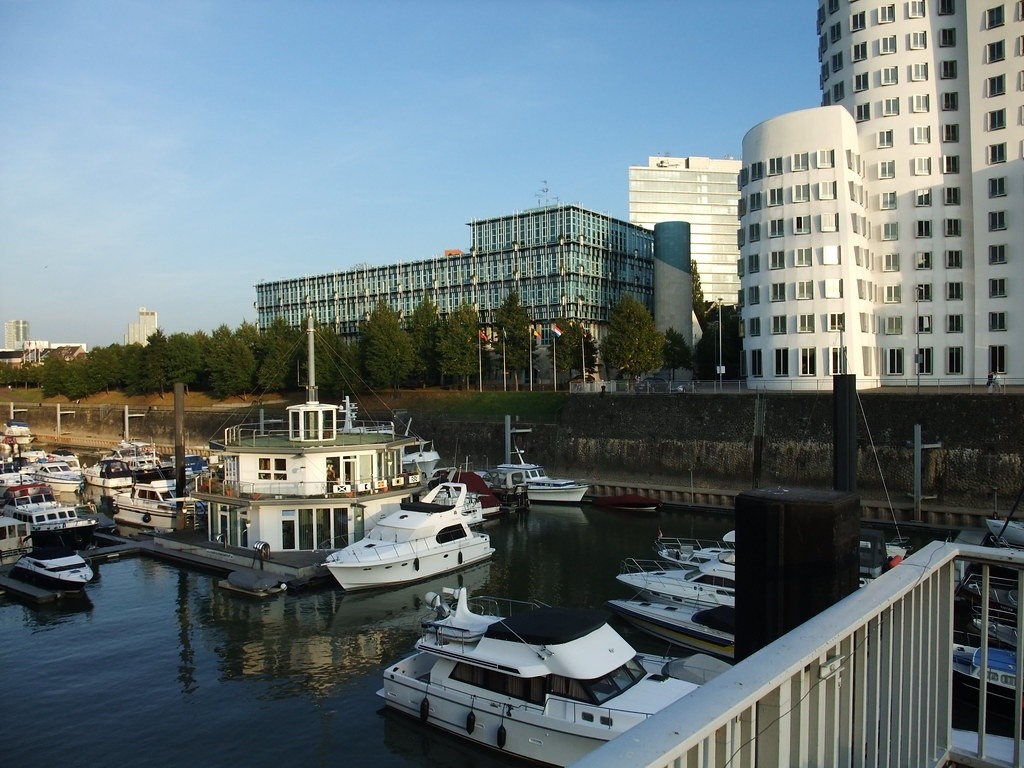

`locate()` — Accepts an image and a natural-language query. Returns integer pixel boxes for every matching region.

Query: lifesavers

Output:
[142,515,152,523]
[111,506,120,515]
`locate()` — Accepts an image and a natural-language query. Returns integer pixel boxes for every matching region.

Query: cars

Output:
[633,376,696,393]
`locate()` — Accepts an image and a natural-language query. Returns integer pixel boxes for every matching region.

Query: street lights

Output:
[717,297,724,392]
[915,286,923,396]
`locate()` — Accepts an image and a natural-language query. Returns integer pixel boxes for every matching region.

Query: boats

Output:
[339,397,443,478]
[595,492,664,513]
[322,482,496,590]
[374,586,703,767]
[606,524,908,662]
[952,515,1024,716]
[480,461,588,503]
[0,421,216,596]
